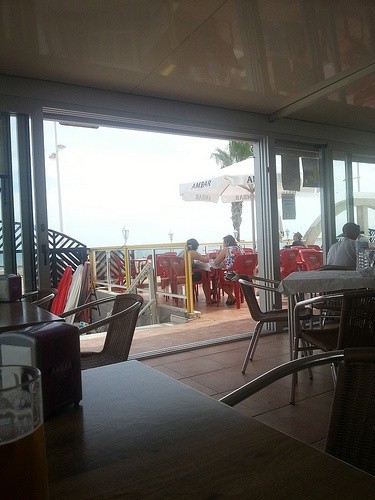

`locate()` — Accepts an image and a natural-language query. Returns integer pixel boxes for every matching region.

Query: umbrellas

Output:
[179,156,320,295]
[50,264,83,324]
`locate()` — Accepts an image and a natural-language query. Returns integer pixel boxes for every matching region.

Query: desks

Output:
[278,269,375,361]
[192,259,238,303]
[0,360,375,500]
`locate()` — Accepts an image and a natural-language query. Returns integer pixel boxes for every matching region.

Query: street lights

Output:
[48,143,67,232]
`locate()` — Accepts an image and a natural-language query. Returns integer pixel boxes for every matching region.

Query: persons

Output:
[187,238,216,303]
[233,251,234,252]
[292,232,305,246]
[327,223,368,267]
[214,235,244,304]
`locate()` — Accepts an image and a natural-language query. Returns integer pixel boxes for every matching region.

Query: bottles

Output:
[356,231,370,273]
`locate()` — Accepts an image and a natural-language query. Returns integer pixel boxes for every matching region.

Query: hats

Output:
[342,223,360,237]
[294,232,302,240]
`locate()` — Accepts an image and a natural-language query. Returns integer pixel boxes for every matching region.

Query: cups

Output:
[0,364,51,500]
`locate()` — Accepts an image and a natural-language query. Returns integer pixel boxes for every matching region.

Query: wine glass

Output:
[365,249,375,268]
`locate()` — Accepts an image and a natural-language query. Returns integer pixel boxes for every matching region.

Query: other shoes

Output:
[210,299,218,303]
[231,298,237,304]
[210,289,214,295]
[226,301,232,305]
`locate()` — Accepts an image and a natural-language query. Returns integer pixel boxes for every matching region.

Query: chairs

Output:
[18,241,375,476]
